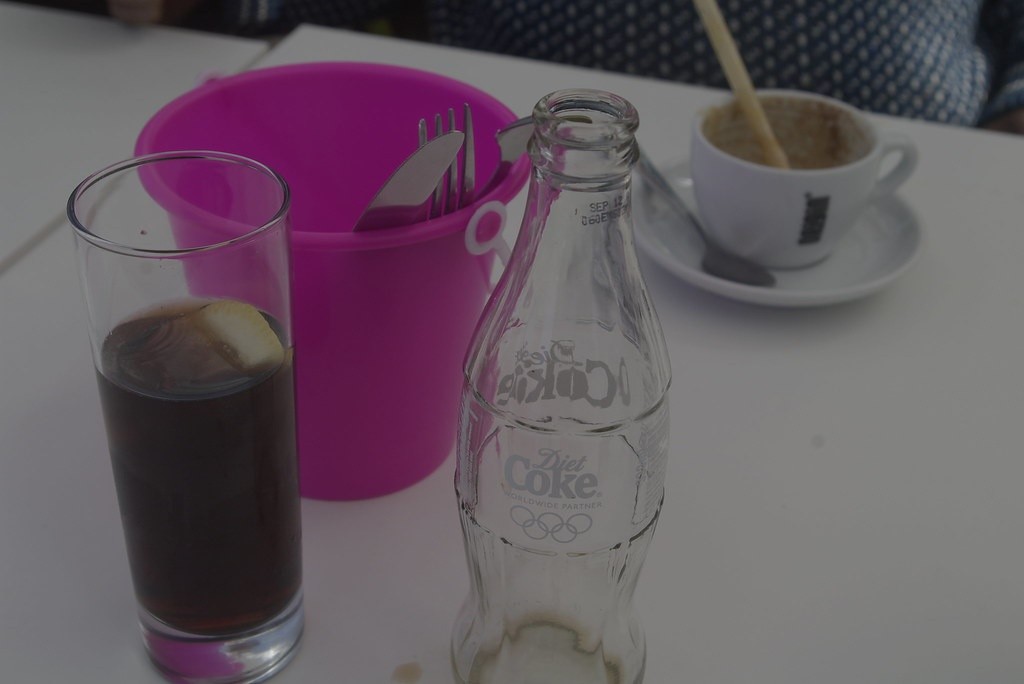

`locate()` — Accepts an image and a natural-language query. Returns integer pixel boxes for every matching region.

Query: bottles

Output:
[448,87,668,684]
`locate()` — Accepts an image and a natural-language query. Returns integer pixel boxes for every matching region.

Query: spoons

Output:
[632,150,779,289]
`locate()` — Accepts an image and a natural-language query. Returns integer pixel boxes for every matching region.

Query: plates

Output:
[628,138,926,309]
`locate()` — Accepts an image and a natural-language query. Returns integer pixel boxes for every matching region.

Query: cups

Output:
[65,150,307,684]
[689,86,918,272]
[130,58,536,502]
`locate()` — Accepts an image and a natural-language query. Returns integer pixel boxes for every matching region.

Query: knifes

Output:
[351,130,465,235]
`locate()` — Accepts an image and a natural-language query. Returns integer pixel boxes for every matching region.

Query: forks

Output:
[416,102,475,221]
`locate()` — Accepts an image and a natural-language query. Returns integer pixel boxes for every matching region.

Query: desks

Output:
[0,0,1024,684]
